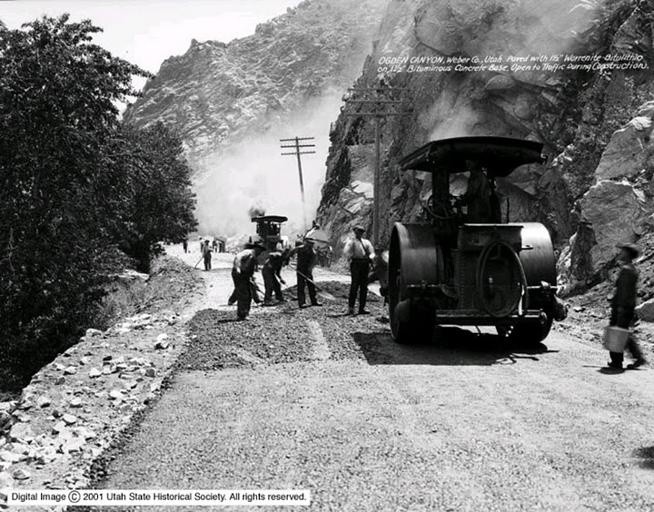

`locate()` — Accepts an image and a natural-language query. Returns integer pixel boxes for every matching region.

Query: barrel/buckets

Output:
[602,324,630,352]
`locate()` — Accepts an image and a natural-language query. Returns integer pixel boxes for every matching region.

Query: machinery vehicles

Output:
[243,213,290,266]
[369,132,570,353]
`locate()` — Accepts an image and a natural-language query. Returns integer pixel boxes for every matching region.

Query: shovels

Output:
[285,284,298,299]
[288,263,336,301]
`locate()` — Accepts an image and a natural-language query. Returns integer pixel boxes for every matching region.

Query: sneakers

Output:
[626,359,644,369]
[227,301,233,305]
[257,299,262,304]
[601,367,621,373]
[348,308,371,315]
[299,300,322,309]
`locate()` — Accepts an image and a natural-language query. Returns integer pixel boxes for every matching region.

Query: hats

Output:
[614,242,640,258]
[305,237,314,244]
[353,225,366,231]
[256,242,267,250]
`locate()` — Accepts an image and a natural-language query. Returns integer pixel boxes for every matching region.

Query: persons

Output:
[199,238,333,320]
[343,225,376,317]
[599,242,646,374]
[183,240,188,253]
